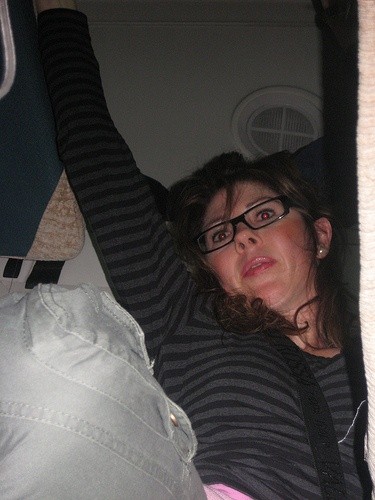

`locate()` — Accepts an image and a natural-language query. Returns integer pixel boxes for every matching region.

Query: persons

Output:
[36,0,375,500]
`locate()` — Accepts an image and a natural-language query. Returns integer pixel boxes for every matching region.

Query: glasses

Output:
[193,195,304,256]
